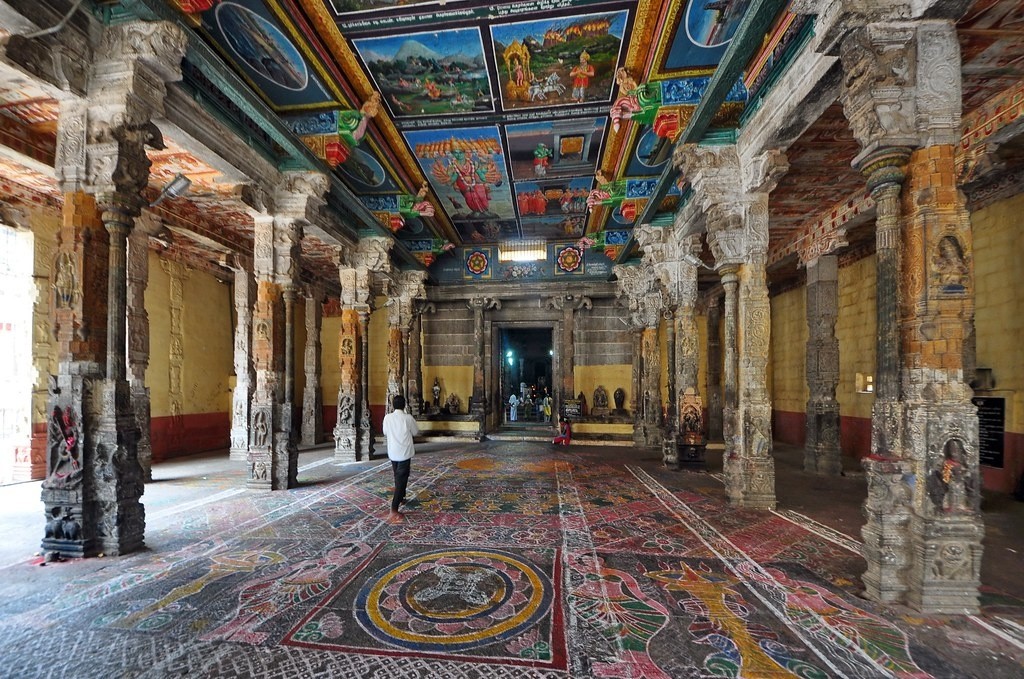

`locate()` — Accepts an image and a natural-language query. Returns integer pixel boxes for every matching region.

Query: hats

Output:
[527,395,530,398]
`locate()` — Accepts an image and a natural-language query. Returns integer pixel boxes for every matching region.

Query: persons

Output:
[508,391,520,421]
[55,254,70,307]
[938,441,973,516]
[383,395,419,516]
[543,392,552,422]
[446,393,460,415]
[937,237,969,284]
[577,391,586,405]
[523,394,534,421]
[432,377,441,405]
[534,393,543,421]
[552,417,571,446]
[594,385,608,406]
[256,413,267,446]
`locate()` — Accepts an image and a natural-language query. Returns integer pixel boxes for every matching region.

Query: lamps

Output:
[149,174,192,207]
[619,316,631,326]
[376,298,394,310]
[683,252,714,271]
